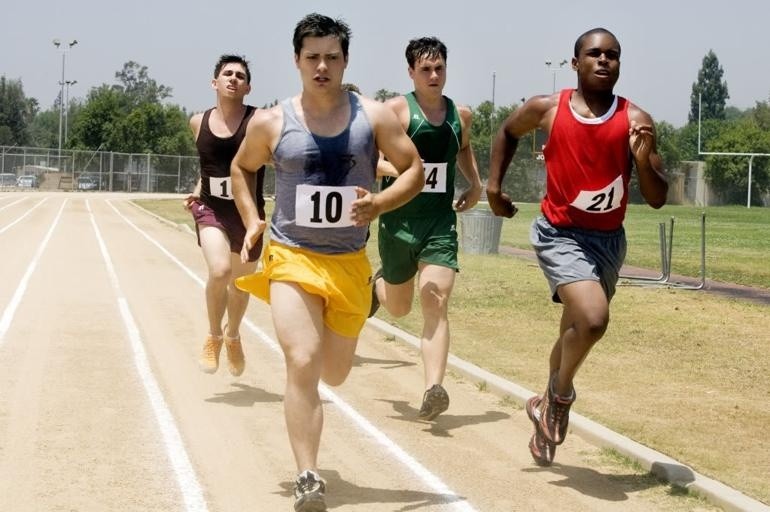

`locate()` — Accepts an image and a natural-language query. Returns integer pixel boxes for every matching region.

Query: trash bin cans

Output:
[456,208,503,255]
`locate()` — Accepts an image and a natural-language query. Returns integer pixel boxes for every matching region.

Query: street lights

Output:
[51,35,78,172]
[58,77,78,175]
[544,58,568,95]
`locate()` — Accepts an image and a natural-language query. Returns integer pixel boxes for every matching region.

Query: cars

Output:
[15,175,39,189]
[76,175,105,191]
[0,172,16,186]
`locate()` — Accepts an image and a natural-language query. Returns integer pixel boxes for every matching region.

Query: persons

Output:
[369,36,485,423]
[230,11,429,512]
[484,27,671,467]
[184,54,281,378]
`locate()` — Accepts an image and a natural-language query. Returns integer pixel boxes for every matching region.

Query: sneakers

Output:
[539,370,575,445]
[419,385,448,421]
[370,268,383,317]
[224,325,245,377]
[526,395,556,466]
[202,334,222,372]
[292,471,328,510]
